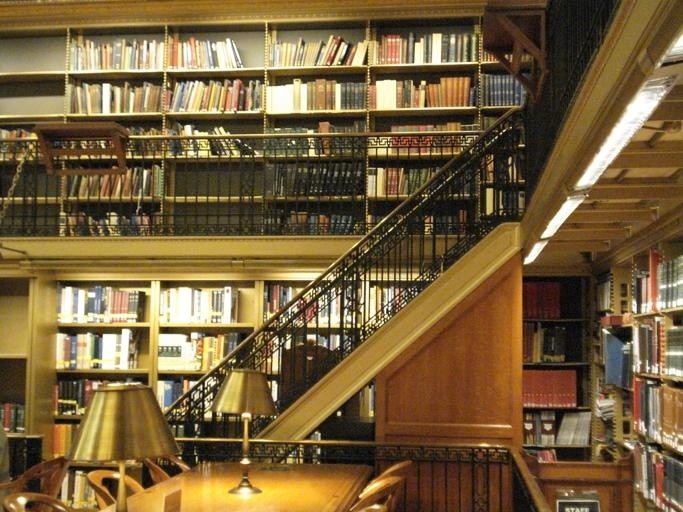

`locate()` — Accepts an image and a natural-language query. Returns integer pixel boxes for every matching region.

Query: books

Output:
[523,256,683,512]
[253,431,321,464]
[0,402,24,434]
[53,376,148,510]
[263,283,423,423]
[63,29,528,234]
[159,287,253,412]
[56,328,140,370]
[168,424,183,451]
[56,282,146,324]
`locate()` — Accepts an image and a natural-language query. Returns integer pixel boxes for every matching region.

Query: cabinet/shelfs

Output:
[0,10,536,232]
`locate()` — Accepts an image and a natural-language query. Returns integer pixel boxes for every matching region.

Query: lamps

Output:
[68,380,177,510]
[206,363,280,493]
